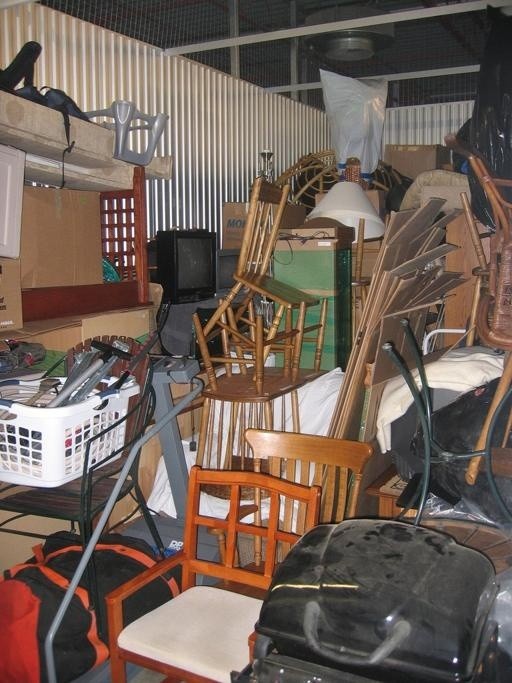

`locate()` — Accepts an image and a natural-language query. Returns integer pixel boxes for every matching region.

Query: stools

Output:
[83,97,171,166]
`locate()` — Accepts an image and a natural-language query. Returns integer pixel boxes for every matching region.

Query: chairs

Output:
[0,133,511,682]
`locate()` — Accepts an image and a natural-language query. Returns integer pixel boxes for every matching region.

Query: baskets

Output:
[0,373,141,490]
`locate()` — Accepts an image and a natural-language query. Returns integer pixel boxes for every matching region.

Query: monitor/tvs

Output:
[146,228,219,303]
